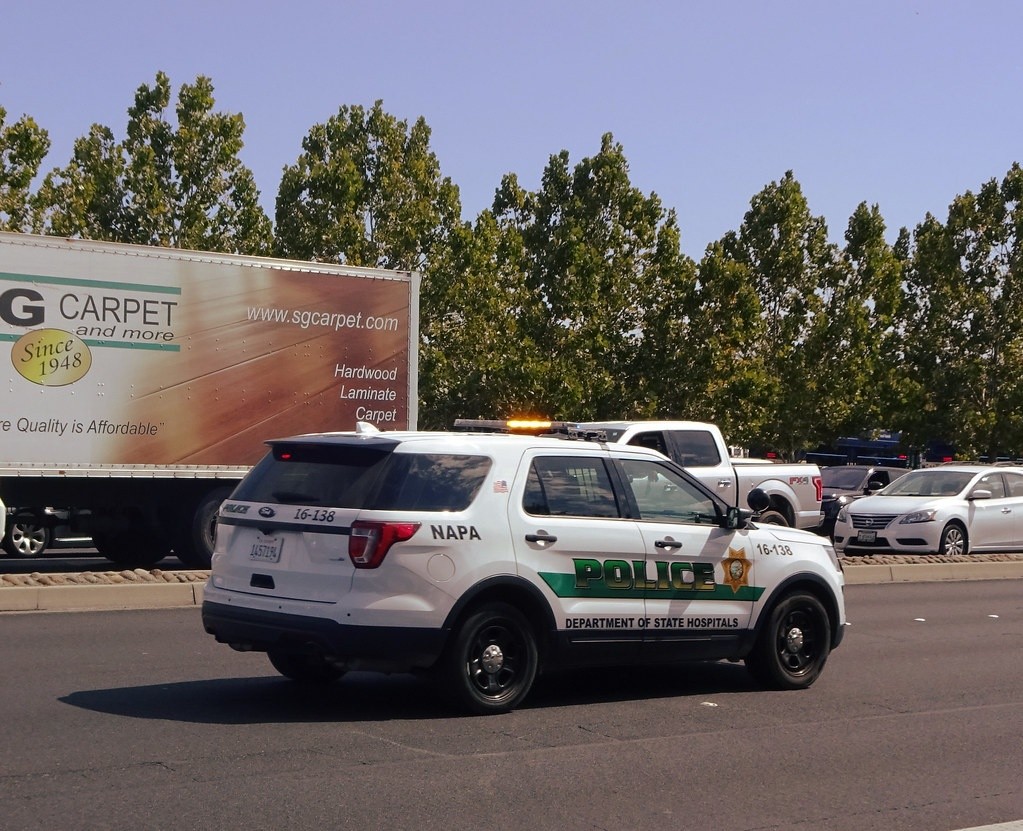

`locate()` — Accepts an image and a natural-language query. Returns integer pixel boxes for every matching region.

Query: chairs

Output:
[552,464,619,518]
[642,439,657,450]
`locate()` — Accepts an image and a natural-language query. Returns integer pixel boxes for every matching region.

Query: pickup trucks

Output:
[554,420,826,532]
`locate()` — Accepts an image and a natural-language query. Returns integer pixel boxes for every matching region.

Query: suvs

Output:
[199,416,849,717]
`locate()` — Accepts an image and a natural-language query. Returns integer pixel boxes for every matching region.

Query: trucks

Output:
[0,231,420,565]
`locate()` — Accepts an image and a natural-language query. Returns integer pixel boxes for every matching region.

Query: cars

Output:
[820,465,913,538]
[0,505,98,561]
[833,465,1023,555]
[813,424,1023,470]
